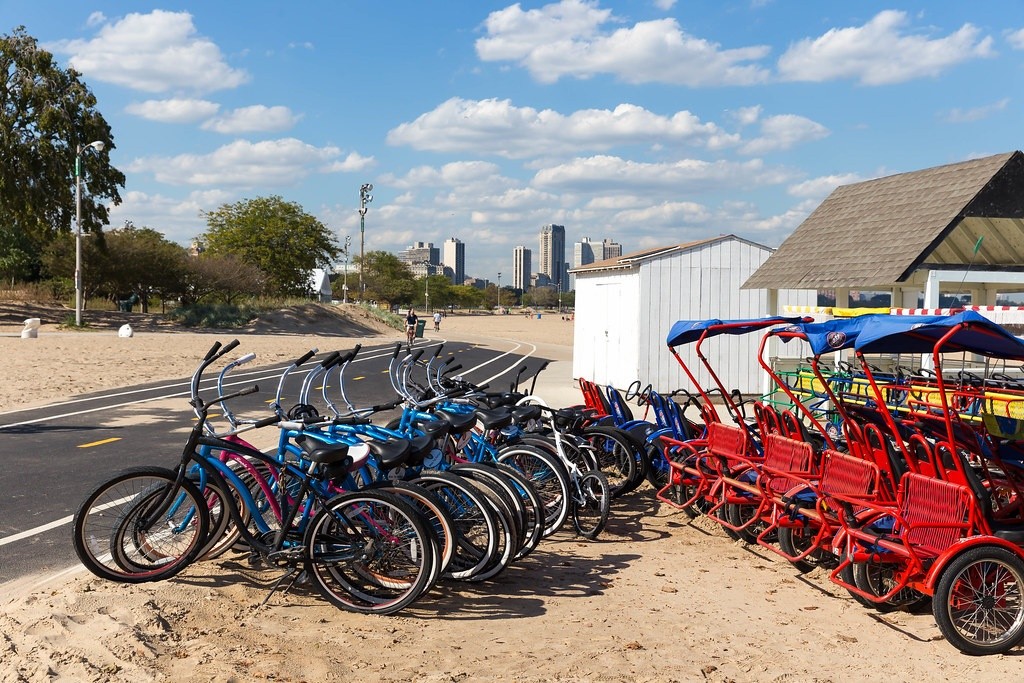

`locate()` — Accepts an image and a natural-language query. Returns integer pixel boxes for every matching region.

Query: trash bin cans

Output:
[415,320,426,338]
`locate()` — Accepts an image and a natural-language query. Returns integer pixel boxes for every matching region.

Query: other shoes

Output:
[406,339,409,343]
[438,327,439,330]
[413,337,415,339]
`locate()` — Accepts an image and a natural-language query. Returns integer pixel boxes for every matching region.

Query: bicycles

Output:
[405,325,415,347]
[71,337,648,618]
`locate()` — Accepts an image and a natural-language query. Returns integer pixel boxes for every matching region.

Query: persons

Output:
[562,312,574,322]
[403,308,418,344]
[503,309,509,314]
[525,313,533,320]
[443,309,446,319]
[433,311,441,331]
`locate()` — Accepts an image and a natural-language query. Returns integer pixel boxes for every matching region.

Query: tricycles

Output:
[656,310,1024,655]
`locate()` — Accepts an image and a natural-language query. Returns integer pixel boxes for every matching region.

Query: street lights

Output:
[343,235,353,304]
[359,184,373,299]
[497,272,501,307]
[75,140,106,327]
[547,283,559,295]
